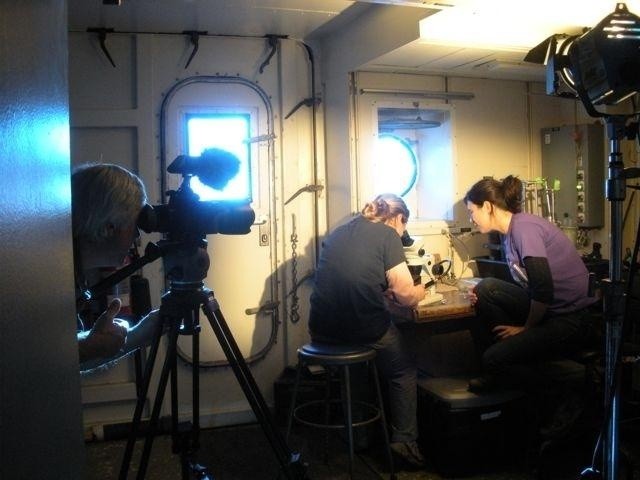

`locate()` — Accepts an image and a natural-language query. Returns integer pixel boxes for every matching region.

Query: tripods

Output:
[116,280,302,480]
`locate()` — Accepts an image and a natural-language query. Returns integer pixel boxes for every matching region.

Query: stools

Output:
[286,345,396,479]
[531,339,635,478]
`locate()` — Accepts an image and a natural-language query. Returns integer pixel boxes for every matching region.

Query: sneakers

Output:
[388,439,428,470]
[468,376,488,391]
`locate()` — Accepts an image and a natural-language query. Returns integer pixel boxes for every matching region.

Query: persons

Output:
[464,175,590,473]
[72,163,149,361]
[308,193,432,467]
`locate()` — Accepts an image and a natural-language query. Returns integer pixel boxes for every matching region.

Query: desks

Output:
[385,274,483,381]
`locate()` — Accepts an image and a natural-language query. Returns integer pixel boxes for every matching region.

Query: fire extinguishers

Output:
[99,247,132,316]
